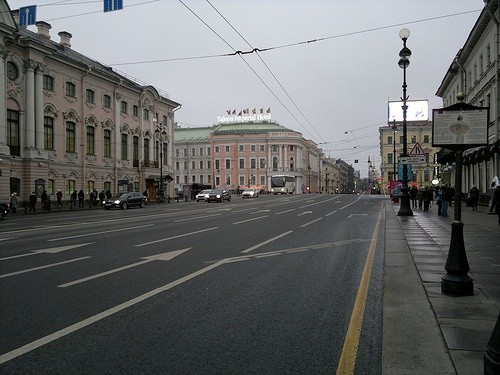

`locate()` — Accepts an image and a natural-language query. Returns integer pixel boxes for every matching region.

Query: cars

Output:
[102,191,148,210]
[195,189,213,202]
[206,188,232,203]
[242,188,259,198]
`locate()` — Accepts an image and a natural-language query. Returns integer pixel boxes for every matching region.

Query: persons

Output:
[370,176,477,220]
[7,181,163,213]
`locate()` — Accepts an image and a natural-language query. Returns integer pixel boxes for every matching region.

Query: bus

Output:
[270,174,296,195]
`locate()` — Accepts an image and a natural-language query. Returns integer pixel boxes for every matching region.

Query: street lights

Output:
[154,123,167,204]
[396,27,415,219]
